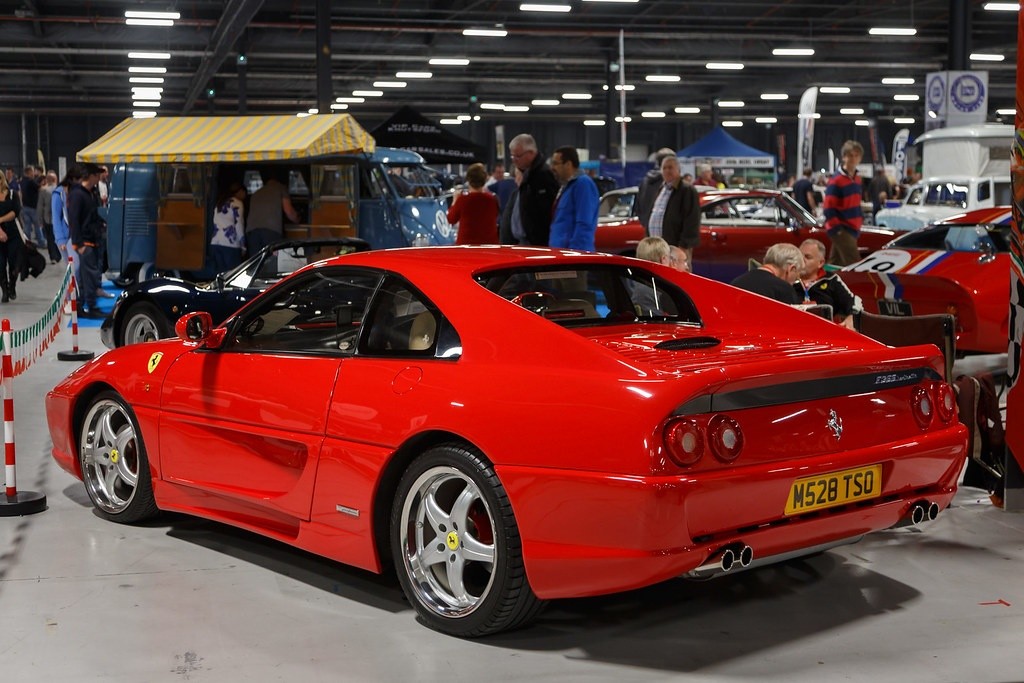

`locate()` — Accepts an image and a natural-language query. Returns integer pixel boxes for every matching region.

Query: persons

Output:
[729,239,854,325]
[245,175,302,259]
[683,163,732,217]
[548,147,600,292]
[51,162,115,319]
[738,140,922,267]
[624,237,689,318]
[631,148,702,273]
[0,163,62,304]
[447,163,500,245]
[509,133,560,287]
[443,176,470,196]
[210,183,247,276]
[482,162,524,245]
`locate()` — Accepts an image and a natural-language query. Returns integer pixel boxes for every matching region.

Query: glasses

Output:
[672,258,688,266]
[549,160,563,167]
[510,150,529,160]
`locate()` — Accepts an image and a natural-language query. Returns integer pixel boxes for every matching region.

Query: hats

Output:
[80,163,105,175]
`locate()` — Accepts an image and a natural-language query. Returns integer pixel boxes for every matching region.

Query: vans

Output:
[76,109,459,293]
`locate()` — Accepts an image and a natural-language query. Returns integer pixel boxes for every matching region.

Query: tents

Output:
[676,125,778,205]
[369,105,489,178]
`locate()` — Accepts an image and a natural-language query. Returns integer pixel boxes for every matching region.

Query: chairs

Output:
[798,305,1004,510]
[409,310,438,351]
[545,298,601,321]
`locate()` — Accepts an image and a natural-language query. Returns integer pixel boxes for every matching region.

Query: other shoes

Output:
[97,288,115,298]
[79,307,108,319]
[2,291,9,303]
[9,283,17,300]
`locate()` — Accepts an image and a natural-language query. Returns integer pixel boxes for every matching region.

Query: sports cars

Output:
[36,234,973,643]
[96,235,610,363]
[447,164,1010,362]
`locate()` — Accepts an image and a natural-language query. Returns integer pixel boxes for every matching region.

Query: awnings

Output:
[76,113,377,163]
